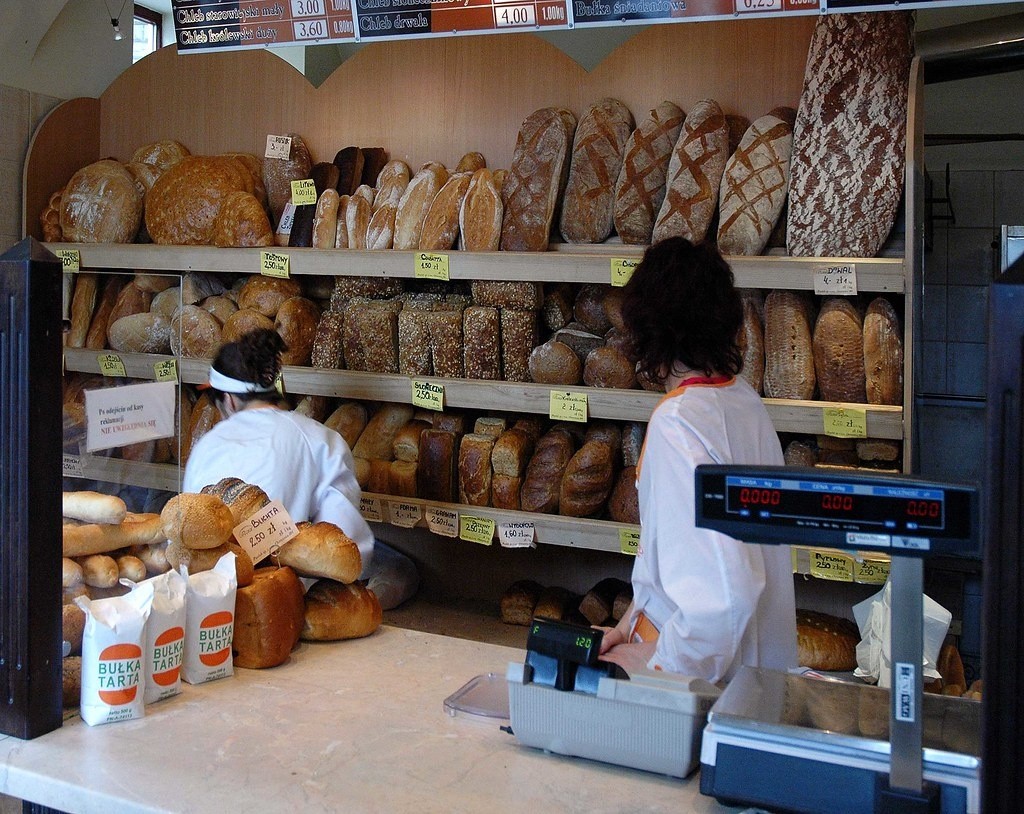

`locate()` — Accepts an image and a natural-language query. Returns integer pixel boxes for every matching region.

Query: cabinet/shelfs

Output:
[20,16,925,685]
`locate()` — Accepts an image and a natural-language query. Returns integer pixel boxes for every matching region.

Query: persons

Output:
[181,330,375,587]
[590,236,798,681]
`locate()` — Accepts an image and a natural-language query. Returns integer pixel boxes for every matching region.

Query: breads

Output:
[40,97,797,257]
[789,0,916,261]
[64,477,384,725]
[65,373,905,552]
[487,538,982,700]
[67,274,901,411]
